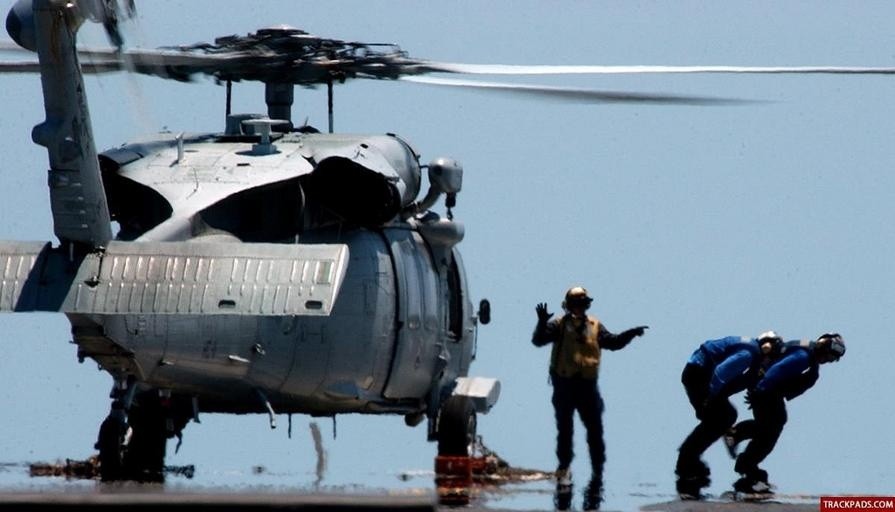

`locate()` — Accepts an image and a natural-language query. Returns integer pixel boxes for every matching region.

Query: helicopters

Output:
[0,0,894,477]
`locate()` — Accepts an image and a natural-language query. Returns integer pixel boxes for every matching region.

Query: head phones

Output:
[562,286,594,311]
[817,333,841,348]
[762,337,782,355]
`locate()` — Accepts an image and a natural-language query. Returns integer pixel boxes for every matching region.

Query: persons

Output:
[722,330,846,491]
[674,330,790,476]
[531,286,650,494]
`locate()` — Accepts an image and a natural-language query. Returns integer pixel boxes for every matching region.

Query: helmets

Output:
[564,286,588,304]
[816,332,847,364]
[758,330,785,355]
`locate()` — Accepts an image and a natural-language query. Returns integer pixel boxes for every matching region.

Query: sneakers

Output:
[674,426,774,475]
[674,476,773,502]
[556,467,604,489]
[554,489,604,510]
